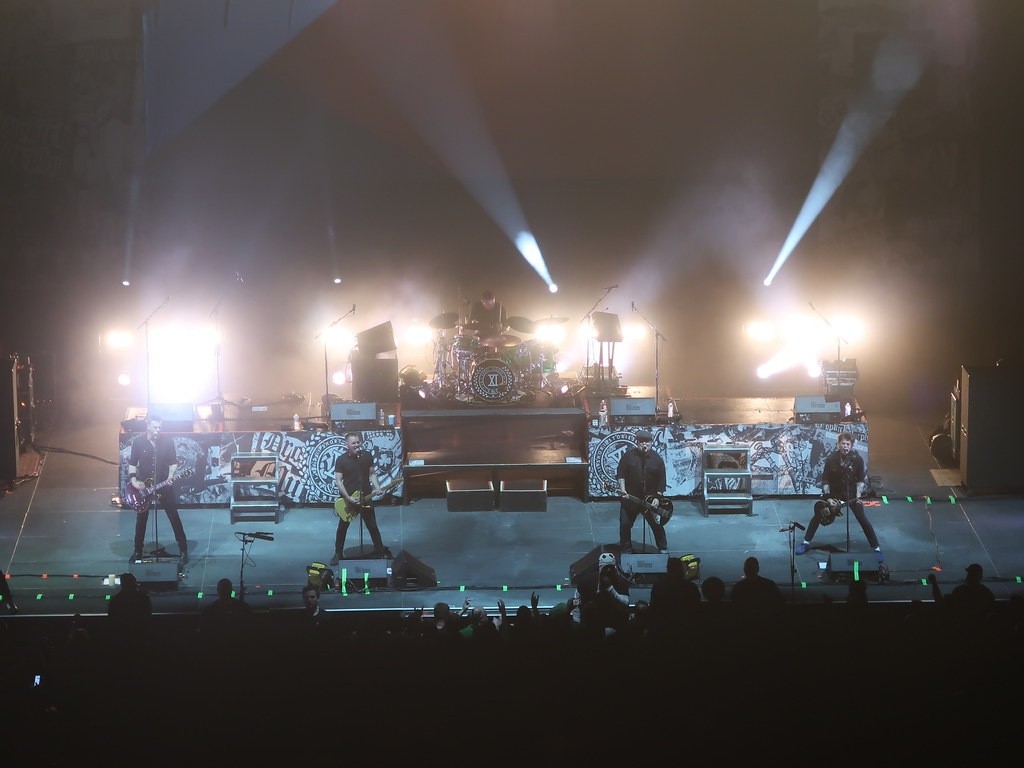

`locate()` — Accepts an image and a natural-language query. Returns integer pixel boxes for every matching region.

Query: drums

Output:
[470,352,516,403]
[516,342,533,357]
[531,344,555,372]
[453,337,480,354]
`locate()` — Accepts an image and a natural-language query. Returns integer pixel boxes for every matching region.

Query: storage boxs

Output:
[354,321,397,354]
[446,480,549,512]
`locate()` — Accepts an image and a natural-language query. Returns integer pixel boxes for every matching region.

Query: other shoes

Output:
[180,547,188,560]
[129,552,141,563]
[795,543,809,556]
[376,545,390,554]
[620,548,630,553]
[660,548,668,553]
[874,549,884,564]
[331,550,342,567]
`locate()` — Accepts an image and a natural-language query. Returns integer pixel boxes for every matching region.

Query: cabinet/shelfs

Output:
[400,406,590,507]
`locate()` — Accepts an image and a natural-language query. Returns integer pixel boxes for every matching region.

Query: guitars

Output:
[334,478,398,523]
[602,480,674,527]
[124,469,178,513]
[814,489,876,526]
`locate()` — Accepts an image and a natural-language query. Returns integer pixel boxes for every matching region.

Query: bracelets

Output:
[130,473,136,479]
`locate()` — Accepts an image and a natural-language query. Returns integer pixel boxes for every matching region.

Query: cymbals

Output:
[534,316,570,323]
[508,316,536,334]
[481,335,520,348]
[429,313,461,328]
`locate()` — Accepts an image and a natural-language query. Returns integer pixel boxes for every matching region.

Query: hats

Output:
[635,431,652,440]
[965,564,983,578]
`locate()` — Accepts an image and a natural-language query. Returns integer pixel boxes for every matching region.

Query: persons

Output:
[468,290,509,338]
[615,431,669,554]
[107,571,152,628]
[330,433,393,565]
[796,432,883,562]
[299,584,328,629]
[128,416,188,565]
[203,578,250,626]
[402,557,996,669]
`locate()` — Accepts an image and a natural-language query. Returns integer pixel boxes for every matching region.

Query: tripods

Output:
[306,309,354,423]
[195,303,240,407]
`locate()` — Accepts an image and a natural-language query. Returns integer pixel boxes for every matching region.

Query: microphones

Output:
[605,285,619,289]
[632,301,634,310]
[790,520,806,531]
[235,531,274,541]
[642,445,647,450]
[153,432,159,437]
[352,304,356,316]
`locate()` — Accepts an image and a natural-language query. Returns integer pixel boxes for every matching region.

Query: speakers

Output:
[129,562,179,593]
[792,395,842,425]
[618,553,669,584]
[391,549,437,592]
[147,403,194,433]
[330,403,380,430]
[609,397,656,426]
[826,552,879,583]
[569,543,622,592]
[340,560,389,590]
[949,361,1024,492]
